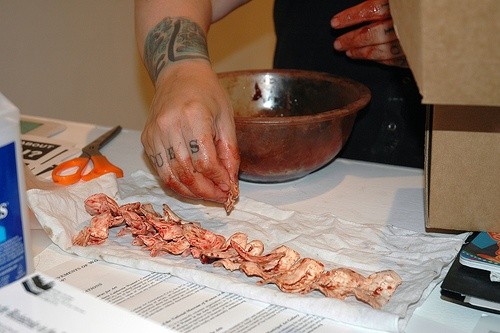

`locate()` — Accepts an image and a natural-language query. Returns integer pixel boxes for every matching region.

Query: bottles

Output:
[0,92,33,287]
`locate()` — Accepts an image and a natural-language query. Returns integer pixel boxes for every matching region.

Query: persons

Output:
[134,0,426,204]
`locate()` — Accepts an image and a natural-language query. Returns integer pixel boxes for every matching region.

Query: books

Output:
[441,230,500,317]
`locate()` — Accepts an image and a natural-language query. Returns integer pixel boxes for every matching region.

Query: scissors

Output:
[51,125,123,185]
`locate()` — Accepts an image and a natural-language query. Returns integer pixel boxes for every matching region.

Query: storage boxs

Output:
[388,0,500,235]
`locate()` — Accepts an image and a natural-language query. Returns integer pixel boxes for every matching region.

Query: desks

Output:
[0,116,500,333]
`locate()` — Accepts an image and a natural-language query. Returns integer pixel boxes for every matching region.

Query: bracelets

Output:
[142,16,212,87]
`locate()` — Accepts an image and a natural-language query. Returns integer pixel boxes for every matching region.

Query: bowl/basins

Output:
[213,68,371,183]
